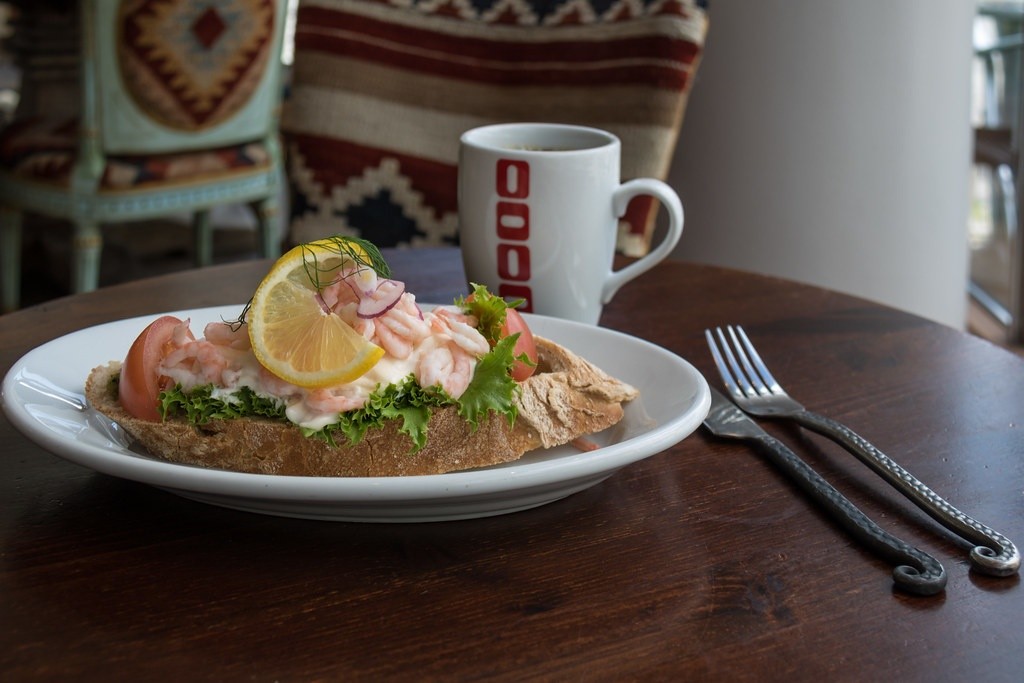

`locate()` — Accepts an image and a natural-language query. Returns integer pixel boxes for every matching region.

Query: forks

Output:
[705,324,1023,578]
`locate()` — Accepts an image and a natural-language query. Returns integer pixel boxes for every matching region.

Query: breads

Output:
[83,336,641,477]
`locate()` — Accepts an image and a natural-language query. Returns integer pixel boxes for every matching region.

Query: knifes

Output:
[703,383,948,597]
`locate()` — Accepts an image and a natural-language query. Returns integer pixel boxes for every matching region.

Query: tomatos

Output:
[117,315,196,423]
[467,292,537,382]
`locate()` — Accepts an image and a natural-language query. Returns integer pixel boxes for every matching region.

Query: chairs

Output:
[277,0,715,259]
[0,0,298,317]
[962,33,1024,343]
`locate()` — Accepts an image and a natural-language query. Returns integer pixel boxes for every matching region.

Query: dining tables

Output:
[0,242,1024,681]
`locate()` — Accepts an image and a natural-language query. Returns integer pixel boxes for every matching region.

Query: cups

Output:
[456,122,684,327]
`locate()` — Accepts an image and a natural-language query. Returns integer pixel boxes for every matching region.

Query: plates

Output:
[0,300,713,522]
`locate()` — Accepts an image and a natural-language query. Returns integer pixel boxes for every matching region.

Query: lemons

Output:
[246,238,386,388]
